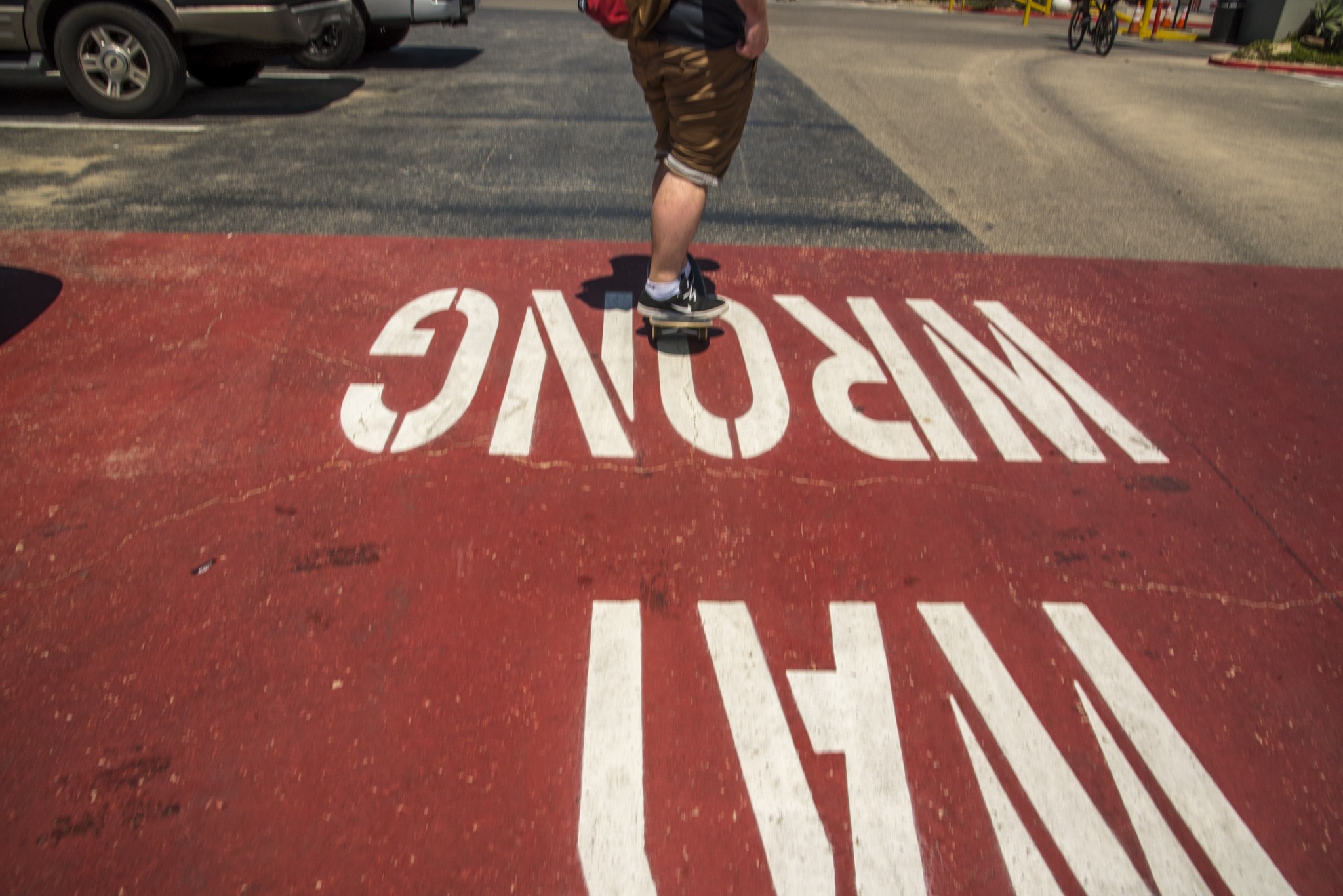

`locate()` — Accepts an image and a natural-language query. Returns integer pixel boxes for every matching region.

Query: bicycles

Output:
[1068,0,1118,56]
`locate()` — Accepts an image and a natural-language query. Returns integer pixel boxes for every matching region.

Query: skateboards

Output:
[647,252,713,342]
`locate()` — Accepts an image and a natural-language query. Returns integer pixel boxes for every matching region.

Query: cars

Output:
[0,1,478,121]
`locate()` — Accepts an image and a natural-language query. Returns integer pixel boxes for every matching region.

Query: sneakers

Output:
[638,273,728,321]
[683,265,693,285]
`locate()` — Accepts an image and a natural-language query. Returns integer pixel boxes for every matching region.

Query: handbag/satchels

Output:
[576,0,632,41]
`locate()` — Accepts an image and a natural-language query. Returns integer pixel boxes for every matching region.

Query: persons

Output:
[626,0,770,321]
